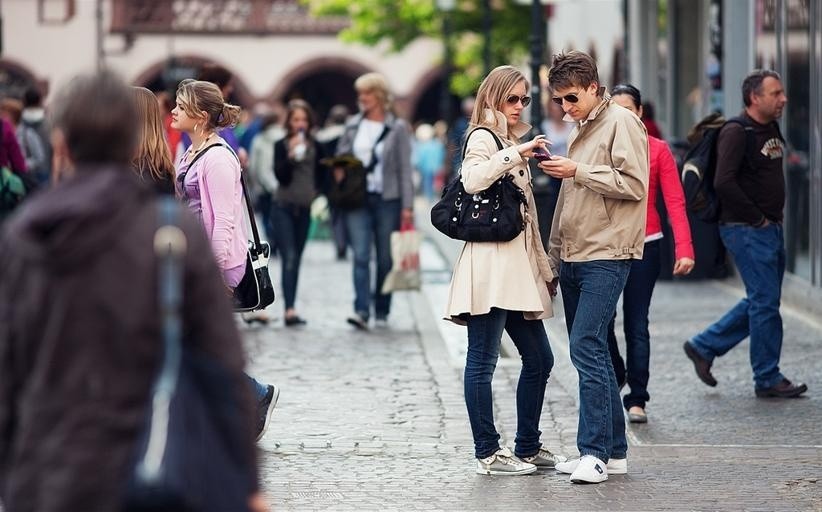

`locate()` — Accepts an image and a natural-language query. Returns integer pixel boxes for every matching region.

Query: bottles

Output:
[295,128,306,162]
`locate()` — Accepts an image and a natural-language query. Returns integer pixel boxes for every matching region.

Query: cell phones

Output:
[534,153,552,163]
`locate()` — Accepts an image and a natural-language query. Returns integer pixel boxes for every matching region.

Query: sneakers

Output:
[347,313,369,329]
[519,450,567,468]
[253,383,278,444]
[478,449,538,477]
[286,316,306,327]
[572,454,609,483]
[558,456,627,478]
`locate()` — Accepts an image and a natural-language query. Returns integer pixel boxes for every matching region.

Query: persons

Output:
[533,50,652,485]
[680,64,812,398]
[431,63,568,481]
[1,61,486,335]
[1,71,263,507]
[168,80,281,446]
[605,79,696,428]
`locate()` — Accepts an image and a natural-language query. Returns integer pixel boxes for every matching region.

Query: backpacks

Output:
[682,120,754,222]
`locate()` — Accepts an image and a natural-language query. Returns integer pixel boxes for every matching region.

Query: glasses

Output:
[553,89,585,104]
[507,96,533,107]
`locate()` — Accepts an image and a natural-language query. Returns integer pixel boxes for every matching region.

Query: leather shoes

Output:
[683,340,717,386]
[626,406,648,422]
[755,377,807,399]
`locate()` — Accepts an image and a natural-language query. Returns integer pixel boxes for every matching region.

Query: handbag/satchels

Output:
[319,156,366,206]
[380,230,422,292]
[431,172,530,241]
[228,241,273,311]
